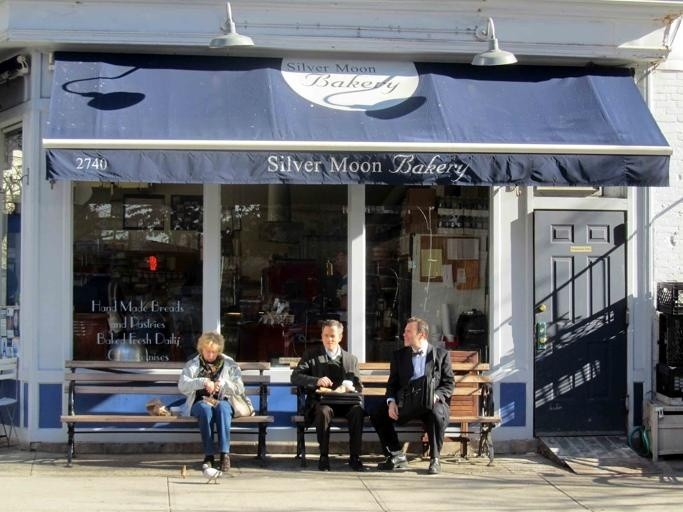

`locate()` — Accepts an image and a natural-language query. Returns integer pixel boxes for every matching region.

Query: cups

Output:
[341,379,354,389]
[428,302,454,343]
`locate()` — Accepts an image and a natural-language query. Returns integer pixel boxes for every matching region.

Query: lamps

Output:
[208,2,255,49]
[471,17,519,66]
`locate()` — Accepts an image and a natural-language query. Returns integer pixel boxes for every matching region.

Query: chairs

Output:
[0,356,23,452]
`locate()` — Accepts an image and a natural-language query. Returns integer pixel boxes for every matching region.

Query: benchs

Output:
[58,356,274,468]
[287,360,502,468]
[236,321,347,362]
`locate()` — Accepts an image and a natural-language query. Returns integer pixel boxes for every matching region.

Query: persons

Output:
[334,249,347,310]
[370,317,455,474]
[169,332,245,472]
[290,319,370,472]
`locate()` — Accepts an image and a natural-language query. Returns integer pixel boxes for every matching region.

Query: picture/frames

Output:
[122,193,165,230]
[170,193,203,231]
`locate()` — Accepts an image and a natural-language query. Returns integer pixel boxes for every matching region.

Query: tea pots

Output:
[107,339,147,363]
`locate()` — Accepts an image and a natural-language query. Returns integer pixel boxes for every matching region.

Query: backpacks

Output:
[456,306,488,349]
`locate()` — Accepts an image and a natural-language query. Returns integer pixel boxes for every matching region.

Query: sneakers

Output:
[202,454,231,472]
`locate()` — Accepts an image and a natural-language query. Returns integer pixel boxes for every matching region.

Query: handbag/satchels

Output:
[318,391,362,405]
[227,384,256,418]
[395,374,435,426]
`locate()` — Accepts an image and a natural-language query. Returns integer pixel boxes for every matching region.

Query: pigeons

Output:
[202,463,222,484]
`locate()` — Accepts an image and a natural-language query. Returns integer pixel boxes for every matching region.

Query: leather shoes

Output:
[348,456,371,472]
[318,454,331,471]
[377,452,409,470]
[427,456,442,473]
